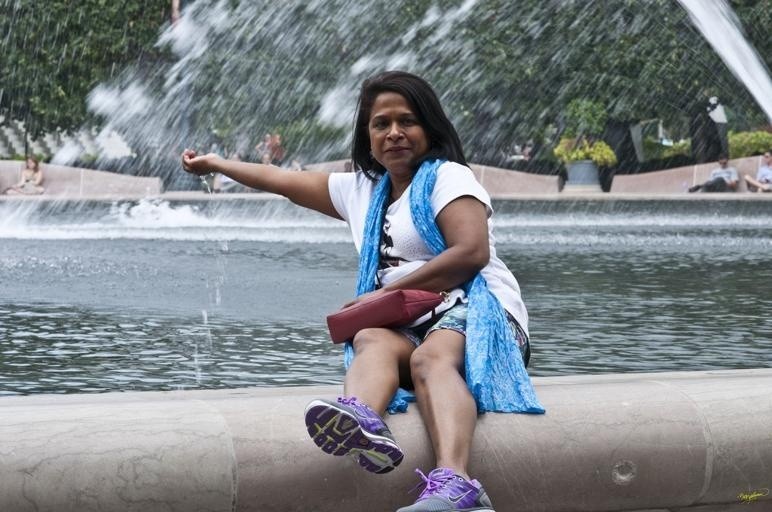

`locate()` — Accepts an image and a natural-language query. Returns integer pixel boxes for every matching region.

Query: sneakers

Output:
[305,397,403,473]
[397,468,495,512]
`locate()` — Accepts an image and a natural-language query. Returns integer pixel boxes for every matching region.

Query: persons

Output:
[3,153,52,196]
[201,133,286,194]
[181,69,532,512]
[688,152,742,195]
[745,146,772,193]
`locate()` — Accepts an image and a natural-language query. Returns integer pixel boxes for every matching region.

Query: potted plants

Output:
[553,137,617,195]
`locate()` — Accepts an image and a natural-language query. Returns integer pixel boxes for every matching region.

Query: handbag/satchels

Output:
[327,289,441,344]
